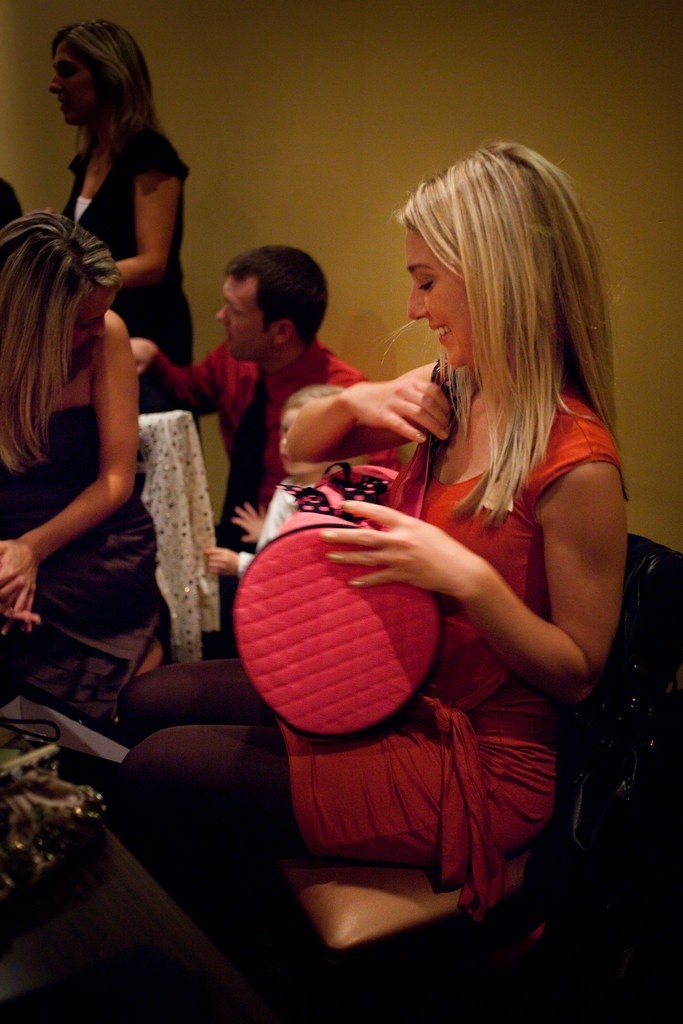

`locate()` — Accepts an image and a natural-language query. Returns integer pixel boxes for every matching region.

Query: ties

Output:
[214,375,270,553]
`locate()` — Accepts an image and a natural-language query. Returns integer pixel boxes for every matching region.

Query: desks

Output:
[0,821,285,1024]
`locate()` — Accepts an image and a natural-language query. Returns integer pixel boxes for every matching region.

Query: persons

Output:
[0,15,373,705]
[112,139,632,1017]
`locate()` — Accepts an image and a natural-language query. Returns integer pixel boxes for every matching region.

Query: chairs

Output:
[270,548,662,1024]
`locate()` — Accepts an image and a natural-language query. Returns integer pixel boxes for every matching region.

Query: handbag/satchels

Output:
[0,767,109,954]
[231,461,446,744]
